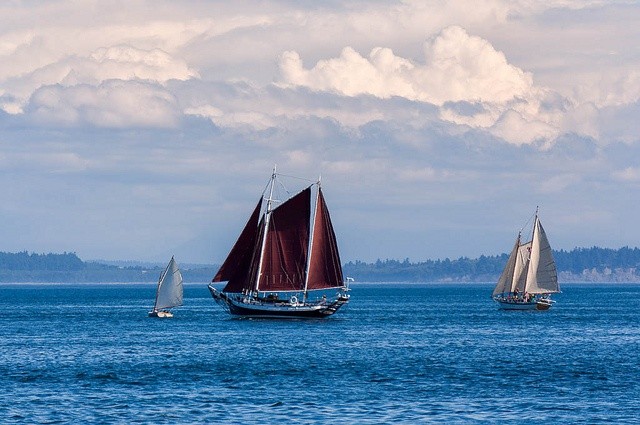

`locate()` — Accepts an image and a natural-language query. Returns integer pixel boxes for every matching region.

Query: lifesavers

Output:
[291,296,297,304]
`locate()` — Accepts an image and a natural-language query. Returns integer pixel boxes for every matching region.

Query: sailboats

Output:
[207,164,354,318]
[148,254,184,318]
[490,205,562,310]
